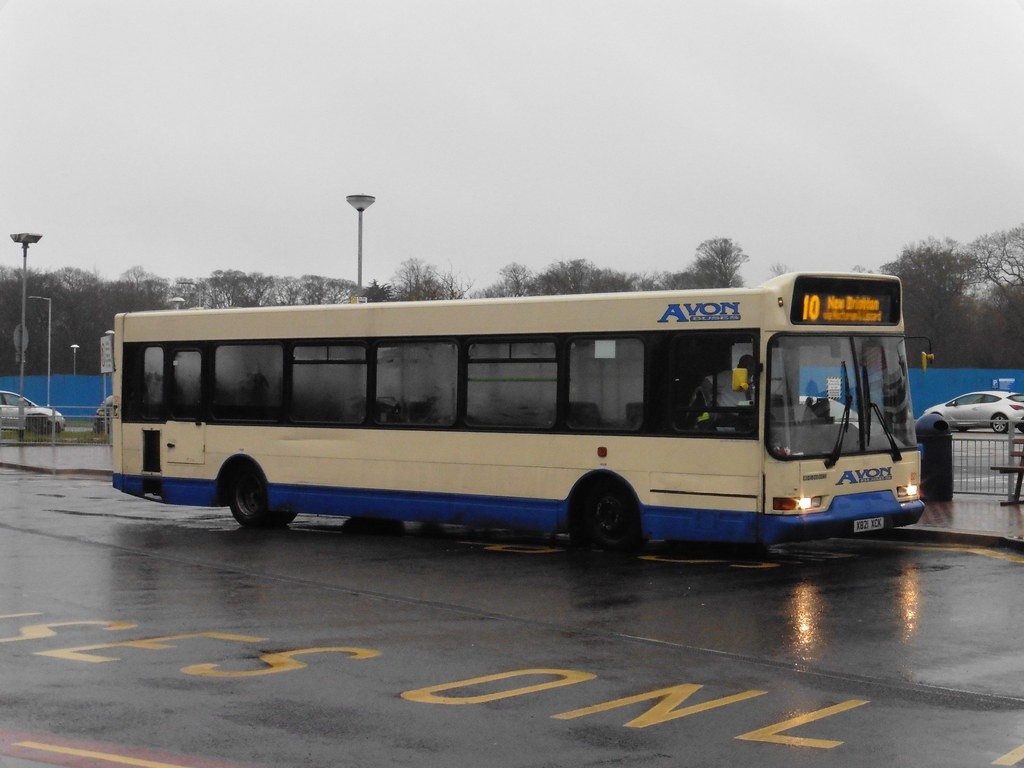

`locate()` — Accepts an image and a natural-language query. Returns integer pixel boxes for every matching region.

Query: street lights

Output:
[9,232,44,441]
[28,295,52,409]
[71,344,80,374]
[346,194,376,302]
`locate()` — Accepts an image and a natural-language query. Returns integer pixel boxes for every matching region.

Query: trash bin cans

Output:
[915,413,954,502]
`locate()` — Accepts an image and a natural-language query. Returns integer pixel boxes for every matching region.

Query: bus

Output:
[108,271,926,560]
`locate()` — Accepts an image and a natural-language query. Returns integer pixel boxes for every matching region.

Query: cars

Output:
[797,396,906,452]
[923,390,1024,434]
[0,390,67,433]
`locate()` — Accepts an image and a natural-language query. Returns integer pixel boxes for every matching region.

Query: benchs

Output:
[547,401,601,430]
[626,401,644,429]
[990,438,1024,506]
[396,401,429,423]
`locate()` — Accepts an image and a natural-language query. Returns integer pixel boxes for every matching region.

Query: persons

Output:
[702,354,756,431]
